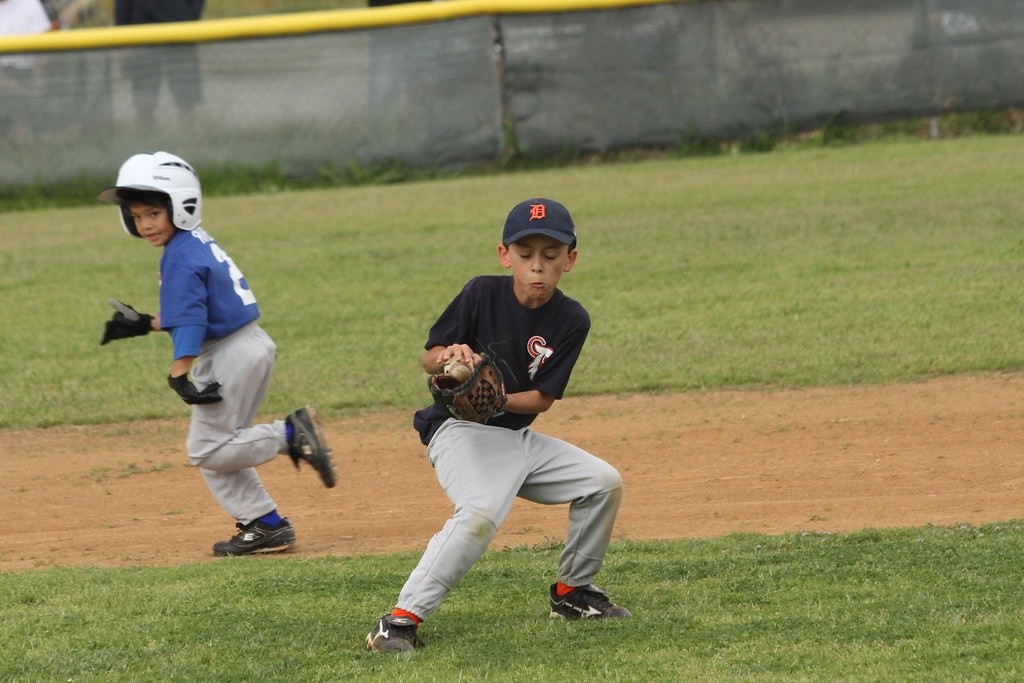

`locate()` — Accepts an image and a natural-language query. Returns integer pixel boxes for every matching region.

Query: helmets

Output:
[98,151,202,239]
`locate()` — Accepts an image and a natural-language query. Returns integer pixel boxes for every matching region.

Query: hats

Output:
[503,198,578,253]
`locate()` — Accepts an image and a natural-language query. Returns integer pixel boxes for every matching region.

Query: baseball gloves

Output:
[427,348,505,423]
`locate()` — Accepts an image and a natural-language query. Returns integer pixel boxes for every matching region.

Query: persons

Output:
[113,0,206,132]
[99,151,337,555]
[366,197,632,653]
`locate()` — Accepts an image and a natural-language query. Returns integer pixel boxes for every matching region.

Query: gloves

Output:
[100,300,155,346]
[168,373,222,405]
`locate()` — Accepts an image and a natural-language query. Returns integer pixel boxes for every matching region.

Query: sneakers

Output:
[549,582,631,624]
[213,516,296,557]
[365,614,424,654]
[287,405,336,489]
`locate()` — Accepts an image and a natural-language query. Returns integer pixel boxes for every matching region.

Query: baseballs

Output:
[443,354,475,382]
